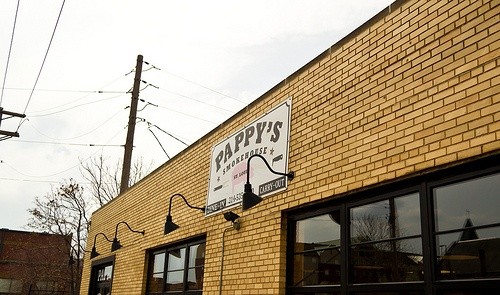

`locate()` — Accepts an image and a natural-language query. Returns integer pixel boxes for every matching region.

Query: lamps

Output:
[163,194,206,236]
[111,221,145,252]
[68,244,91,265]
[90,233,120,260]
[242,154,295,211]
